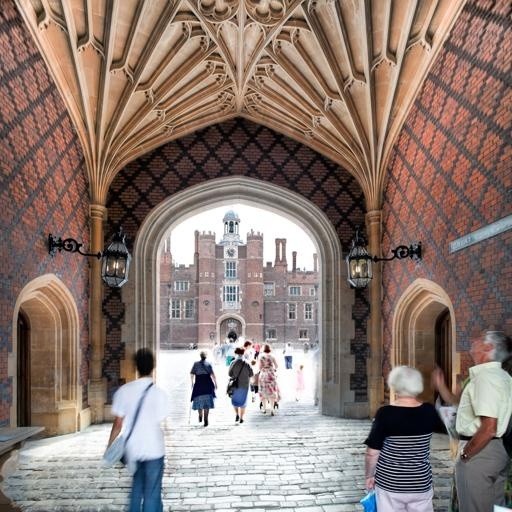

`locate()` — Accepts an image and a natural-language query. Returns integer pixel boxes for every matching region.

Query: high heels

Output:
[199,417,208,427]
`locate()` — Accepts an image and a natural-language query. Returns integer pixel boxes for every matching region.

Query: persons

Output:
[105,348,173,512]
[294,365,304,400]
[431,330,512,512]
[283,342,293,369]
[214,338,261,373]
[257,345,278,416]
[303,341,310,353]
[189,341,194,349]
[363,365,448,512]
[189,351,217,427]
[228,348,254,423]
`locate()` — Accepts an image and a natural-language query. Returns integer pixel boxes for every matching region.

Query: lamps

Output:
[47,225,134,292]
[342,227,420,291]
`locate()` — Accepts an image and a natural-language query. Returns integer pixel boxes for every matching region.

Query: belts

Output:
[459,434,500,440]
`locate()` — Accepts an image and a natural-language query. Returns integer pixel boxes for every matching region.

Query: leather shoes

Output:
[235,416,244,423]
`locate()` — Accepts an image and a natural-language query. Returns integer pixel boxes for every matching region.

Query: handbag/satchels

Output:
[227,380,239,390]
[359,490,377,512]
[97,432,130,470]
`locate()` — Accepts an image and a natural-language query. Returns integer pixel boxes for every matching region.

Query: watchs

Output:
[460,450,471,461]
